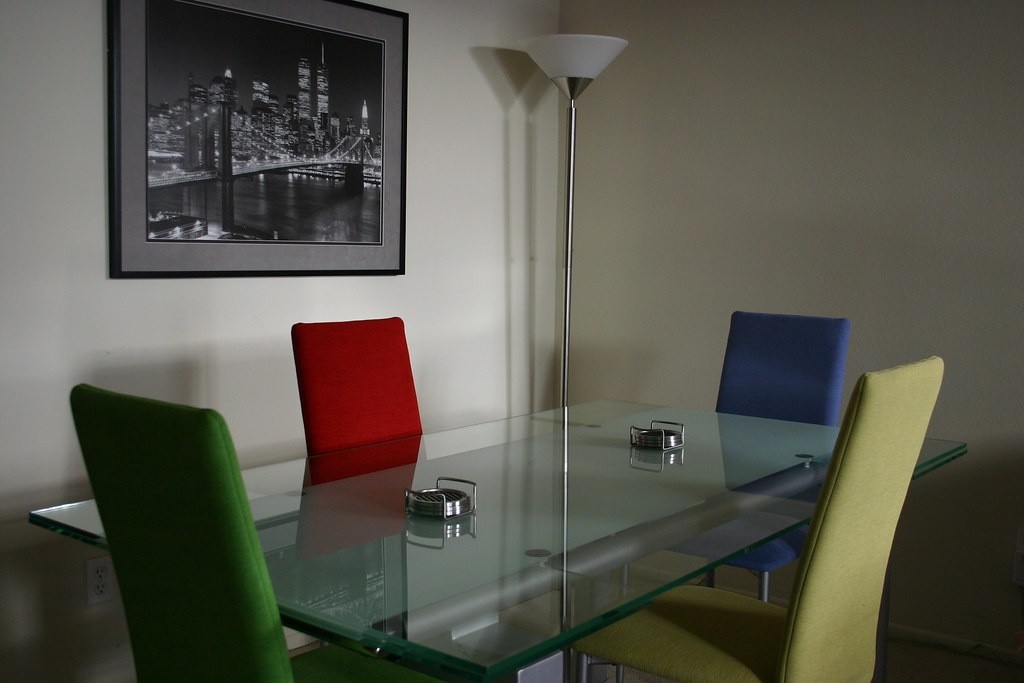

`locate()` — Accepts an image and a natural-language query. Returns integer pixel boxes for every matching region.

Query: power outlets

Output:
[85,554,112,607]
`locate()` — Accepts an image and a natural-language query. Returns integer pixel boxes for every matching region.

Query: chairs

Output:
[291,317,423,458]
[69,383,448,682]
[666,310,849,603]
[717,412,831,504]
[295,434,422,555]
[563,355,945,682]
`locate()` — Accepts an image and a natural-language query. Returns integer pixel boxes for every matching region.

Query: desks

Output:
[28,399,970,683]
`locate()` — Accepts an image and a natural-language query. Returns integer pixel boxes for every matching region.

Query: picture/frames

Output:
[106,0,410,279]
[251,511,408,643]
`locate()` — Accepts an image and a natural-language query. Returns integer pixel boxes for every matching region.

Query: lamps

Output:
[522,35,628,683]
